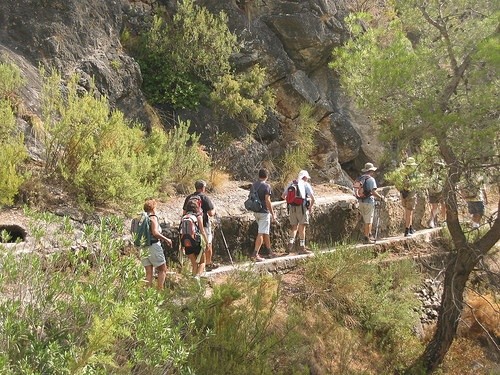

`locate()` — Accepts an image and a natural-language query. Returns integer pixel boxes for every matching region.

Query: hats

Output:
[404,157,417,165]
[298,170,311,180]
[360,163,378,172]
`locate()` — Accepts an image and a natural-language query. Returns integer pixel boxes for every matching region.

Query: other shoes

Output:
[205,263,219,271]
[251,256,265,262]
[364,236,376,244]
[267,252,275,259]
[299,247,311,254]
[285,244,292,253]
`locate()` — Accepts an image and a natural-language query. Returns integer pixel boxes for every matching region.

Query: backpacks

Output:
[287,181,303,207]
[244,186,262,211]
[136,214,157,246]
[353,177,372,199]
[429,180,443,194]
[180,215,199,247]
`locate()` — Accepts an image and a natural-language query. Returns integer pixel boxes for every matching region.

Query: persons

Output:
[250,168,278,262]
[137,199,172,289]
[359,163,384,244]
[180,180,220,281]
[282,169,315,254]
[401,157,490,238]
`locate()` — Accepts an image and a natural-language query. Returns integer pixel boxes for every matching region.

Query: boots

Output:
[404,229,410,237]
[410,226,413,234]
